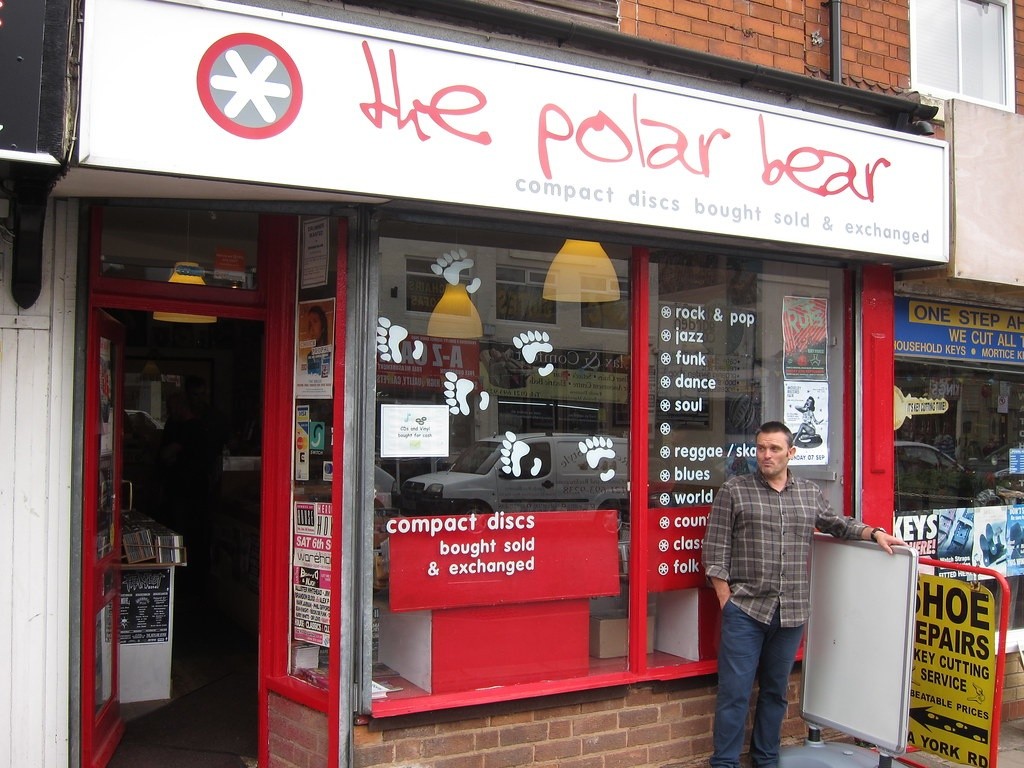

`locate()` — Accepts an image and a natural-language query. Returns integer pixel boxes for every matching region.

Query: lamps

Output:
[542,239,621,302]
[426,227,484,339]
[153,212,218,323]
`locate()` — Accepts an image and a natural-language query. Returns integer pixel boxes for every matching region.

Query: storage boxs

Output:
[591,608,658,657]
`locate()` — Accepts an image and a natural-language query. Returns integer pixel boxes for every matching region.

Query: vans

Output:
[399,433,629,537]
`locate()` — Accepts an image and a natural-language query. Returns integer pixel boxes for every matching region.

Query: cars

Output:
[296,462,396,517]
[894,439,966,494]
[960,440,1024,488]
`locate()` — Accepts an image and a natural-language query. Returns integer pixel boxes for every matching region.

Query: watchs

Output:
[871,527,886,542]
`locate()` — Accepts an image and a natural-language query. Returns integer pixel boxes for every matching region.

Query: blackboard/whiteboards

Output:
[798,532,920,756]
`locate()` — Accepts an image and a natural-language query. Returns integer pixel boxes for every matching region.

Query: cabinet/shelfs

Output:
[101,563,175,705]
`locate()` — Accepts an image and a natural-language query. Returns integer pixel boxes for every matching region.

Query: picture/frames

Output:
[104,308,263,433]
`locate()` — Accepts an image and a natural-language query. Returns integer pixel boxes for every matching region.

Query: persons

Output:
[124,376,229,609]
[703,421,908,768]
[308,305,329,373]
